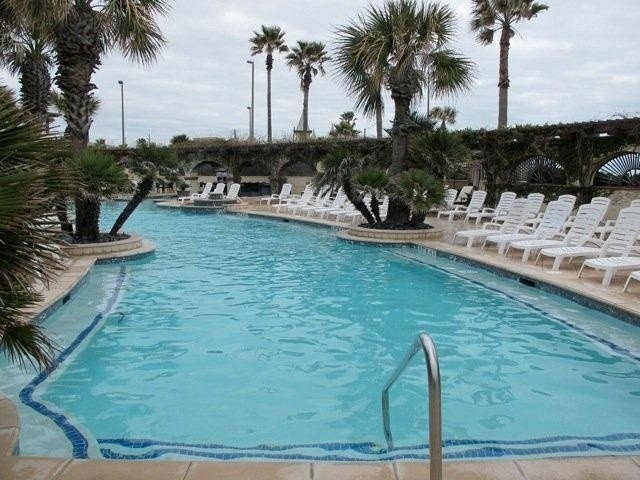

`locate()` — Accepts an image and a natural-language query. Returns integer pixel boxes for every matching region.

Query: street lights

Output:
[246,105,252,140]
[118,80,126,146]
[246,60,257,140]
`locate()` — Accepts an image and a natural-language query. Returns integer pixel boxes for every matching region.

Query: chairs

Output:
[260,183,390,226]
[412,186,640,293]
[180,182,244,205]
